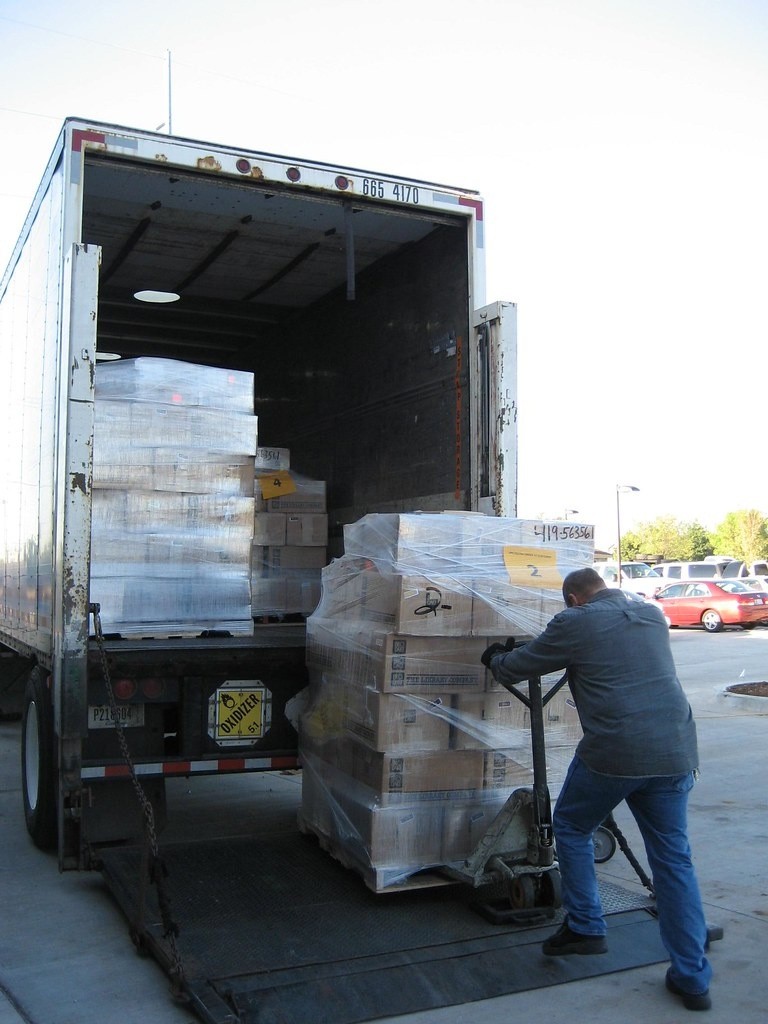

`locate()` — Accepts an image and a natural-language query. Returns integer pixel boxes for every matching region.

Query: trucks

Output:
[0,110,526,874]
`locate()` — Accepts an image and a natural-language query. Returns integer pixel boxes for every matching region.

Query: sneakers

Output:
[667,966,715,1011]
[543,921,609,955]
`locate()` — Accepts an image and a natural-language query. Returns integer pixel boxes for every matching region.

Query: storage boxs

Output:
[91,355,604,896]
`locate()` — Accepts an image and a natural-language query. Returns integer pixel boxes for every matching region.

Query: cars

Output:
[731,575,768,628]
[589,561,665,600]
[645,579,768,633]
[746,558,768,582]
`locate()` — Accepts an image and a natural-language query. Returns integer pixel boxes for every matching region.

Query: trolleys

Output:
[428,636,618,935]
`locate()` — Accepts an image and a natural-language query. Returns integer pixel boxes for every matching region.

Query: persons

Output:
[480,568,716,1011]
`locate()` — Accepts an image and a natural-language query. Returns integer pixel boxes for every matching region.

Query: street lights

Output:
[614,482,641,594]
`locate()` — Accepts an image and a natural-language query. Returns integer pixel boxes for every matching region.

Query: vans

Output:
[702,555,736,565]
[647,563,748,585]
